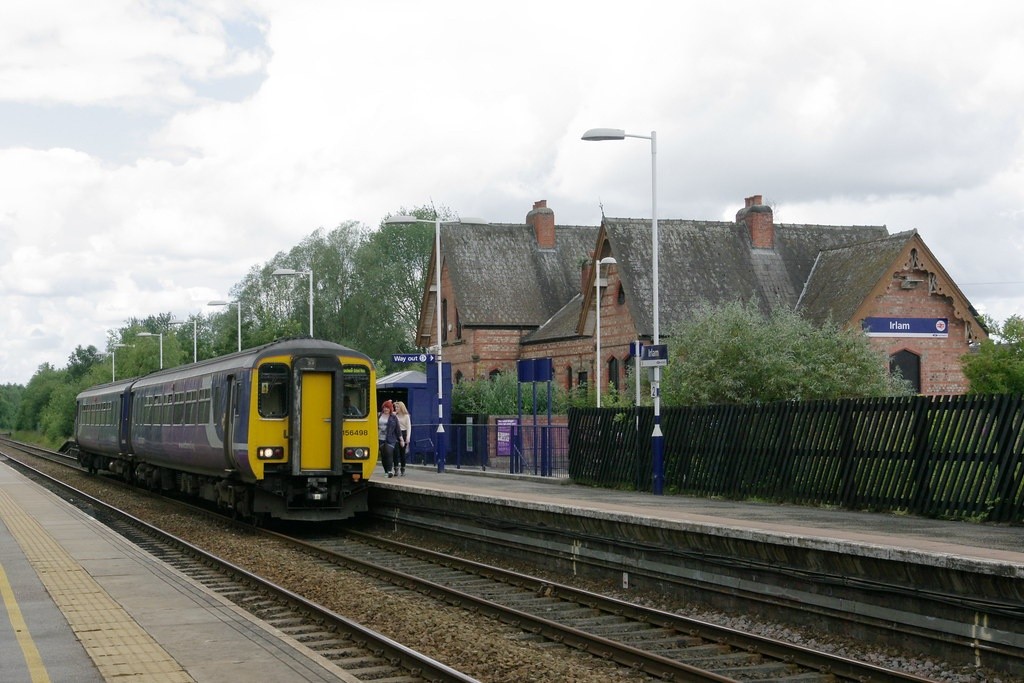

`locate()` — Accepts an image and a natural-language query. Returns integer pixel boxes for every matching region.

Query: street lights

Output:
[168,320,197,363]
[95,351,114,382]
[271,268,313,338]
[137,332,162,369]
[385,216,445,474]
[207,301,241,352]
[582,128,663,494]
[596,257,617,442]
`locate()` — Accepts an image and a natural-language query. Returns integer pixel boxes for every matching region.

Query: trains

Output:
[75,335,379,525]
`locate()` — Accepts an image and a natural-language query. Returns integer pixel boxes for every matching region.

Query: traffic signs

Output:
[391,353,435,363]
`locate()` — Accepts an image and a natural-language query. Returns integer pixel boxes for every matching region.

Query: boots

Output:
[394,468,399,476]
[401,467,405,476]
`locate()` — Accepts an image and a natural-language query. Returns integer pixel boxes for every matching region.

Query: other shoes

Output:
[385,473,388,478]
[388,472,393,478]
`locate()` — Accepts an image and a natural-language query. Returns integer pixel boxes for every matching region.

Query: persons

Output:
[392,401,411,476]
[378,400,405,479]
[344,396,362,415]
